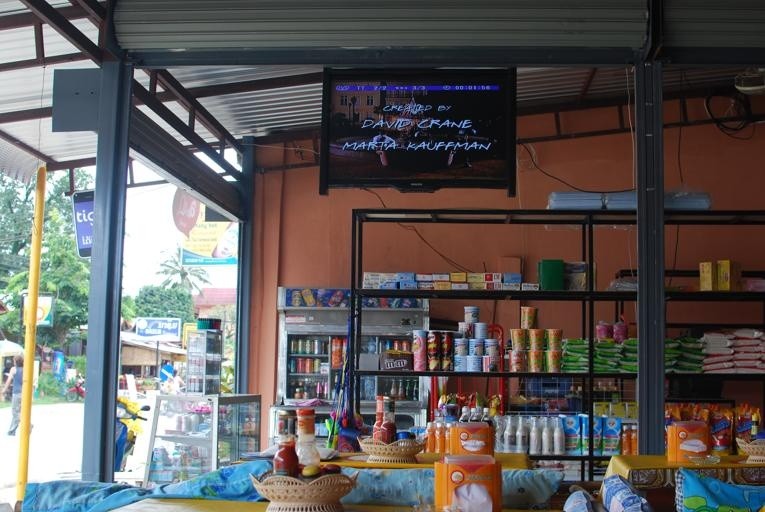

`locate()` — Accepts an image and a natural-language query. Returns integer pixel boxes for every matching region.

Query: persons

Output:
[160,360,174,382]
[0,355,33,436]
[373,129,396,151]
[167,370,187,396]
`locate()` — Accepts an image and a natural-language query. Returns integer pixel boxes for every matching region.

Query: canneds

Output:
[292,289,402,308]
[295,409,315,443]
[375,395,395,422]
[277,410,295,443]
[288,338,412,374]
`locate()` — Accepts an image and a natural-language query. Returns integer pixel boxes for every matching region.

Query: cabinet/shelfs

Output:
[186,328,222,397]
[347,203,594,484]
[268,405,430,448]
[270,284,434,409]
[586,202,765,490]
[143,387,264,488]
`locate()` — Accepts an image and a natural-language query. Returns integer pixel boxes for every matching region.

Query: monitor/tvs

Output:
[319,66,516,198]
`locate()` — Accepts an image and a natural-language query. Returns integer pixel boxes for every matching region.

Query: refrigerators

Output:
[275,286,431,442]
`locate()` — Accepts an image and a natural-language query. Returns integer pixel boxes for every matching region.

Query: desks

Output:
[318,443,540,478]
[599,448,764,491]
[108,492,423,510]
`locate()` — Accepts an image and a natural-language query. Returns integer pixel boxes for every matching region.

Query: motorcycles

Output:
[66,372,85,403]
[114,394,151,473]
[160,386,186,415]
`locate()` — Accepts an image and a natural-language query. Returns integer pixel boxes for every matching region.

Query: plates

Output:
[684,453,721,466]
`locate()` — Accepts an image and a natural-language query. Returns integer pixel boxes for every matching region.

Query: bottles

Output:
[378,378,418,402]
[373,396,383,445]
[287,377,328,398]
[273,408,299,479]
[621,424,638,455]
[750,412,759,440]
[381,395,397,444]
[295,408,321,475]
[426,406,565,455]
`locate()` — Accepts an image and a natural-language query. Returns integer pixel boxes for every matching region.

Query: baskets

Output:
[356,436,427,465]
[249,470,360,512]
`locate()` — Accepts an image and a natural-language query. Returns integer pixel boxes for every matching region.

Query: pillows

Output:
[674,467,765,512]
[602,476,652,509]
[564,490,599,510]
